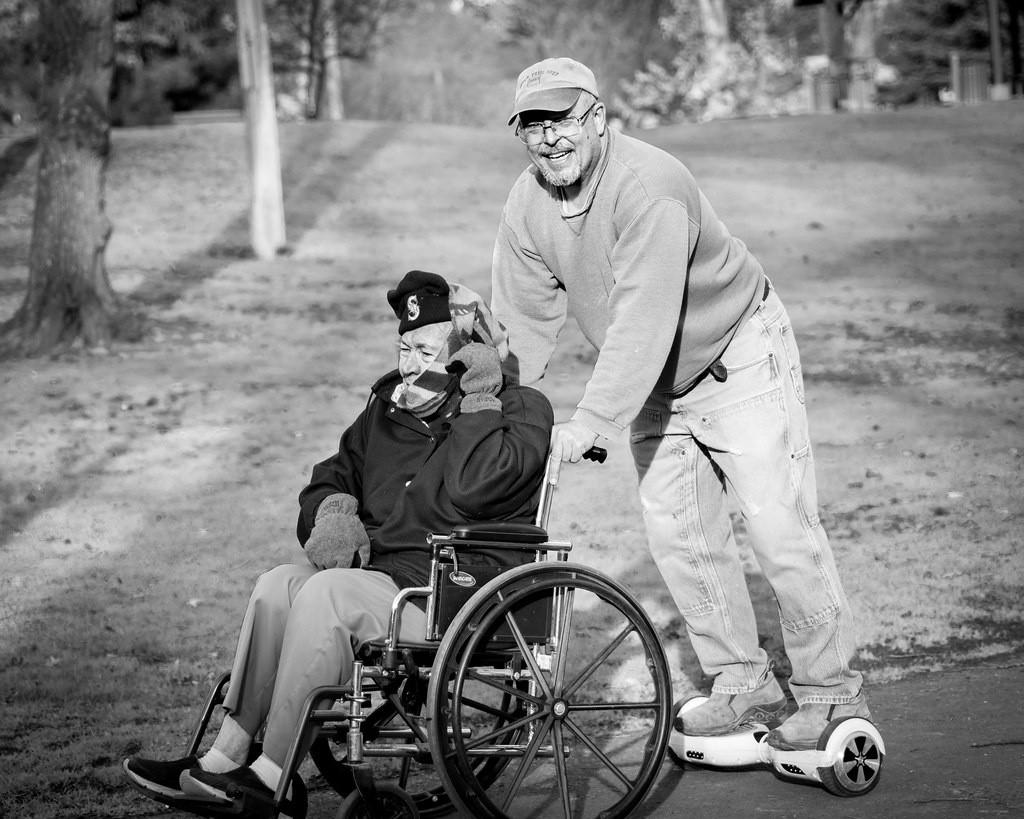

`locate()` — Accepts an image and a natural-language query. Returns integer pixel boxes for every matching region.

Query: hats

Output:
[387,270,451,336]
[507,57,599,126]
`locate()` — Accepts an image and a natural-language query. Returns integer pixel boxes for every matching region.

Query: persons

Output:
[119,272,556,819]
[870,60,905,112]
[487,55,875,749]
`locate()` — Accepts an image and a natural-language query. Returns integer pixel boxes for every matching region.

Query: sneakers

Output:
[766,686,874,750]
[674,658,787,736]
[179,766,293,819]
[123,754,234,808]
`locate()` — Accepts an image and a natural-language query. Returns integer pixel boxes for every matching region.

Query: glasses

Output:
[515,103,595,146]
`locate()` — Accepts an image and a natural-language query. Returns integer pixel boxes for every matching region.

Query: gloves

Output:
[305,493,371,572]
[447,342,503,413]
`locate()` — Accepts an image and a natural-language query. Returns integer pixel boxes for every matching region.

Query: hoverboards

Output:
[672,693,887,795]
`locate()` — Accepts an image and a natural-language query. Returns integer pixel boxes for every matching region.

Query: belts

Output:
[708,277,769,382]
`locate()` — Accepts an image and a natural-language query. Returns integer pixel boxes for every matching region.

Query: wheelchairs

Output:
[129,416,675,819]
